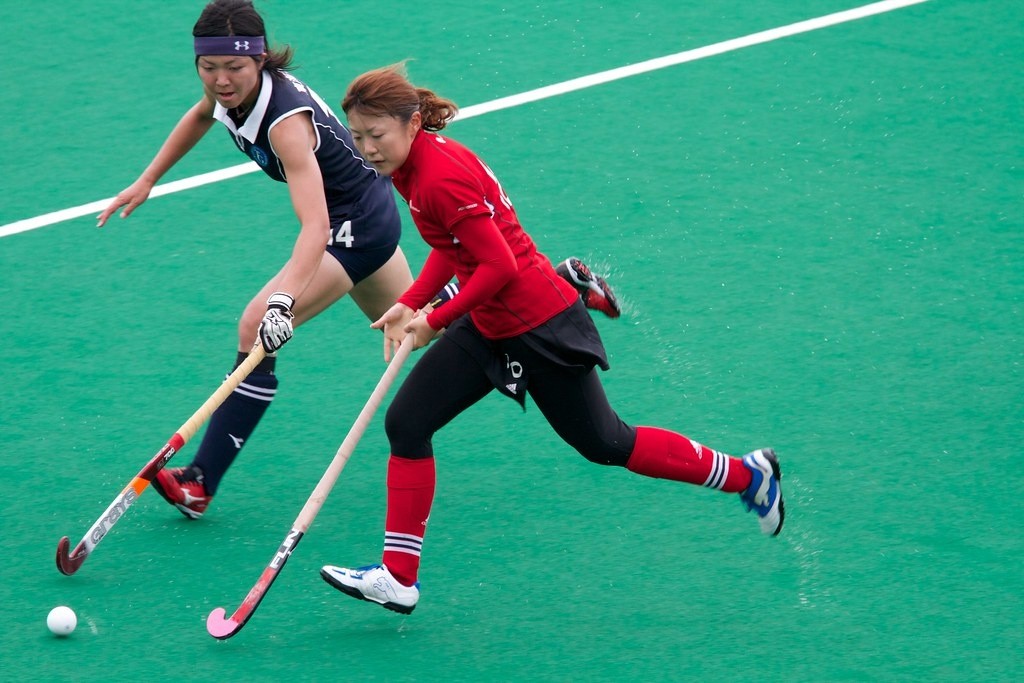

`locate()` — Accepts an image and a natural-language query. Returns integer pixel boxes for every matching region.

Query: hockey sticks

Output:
[54,343,266,576]
[206,307,431,639]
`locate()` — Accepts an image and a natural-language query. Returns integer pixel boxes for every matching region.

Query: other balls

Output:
[47,606,79,637]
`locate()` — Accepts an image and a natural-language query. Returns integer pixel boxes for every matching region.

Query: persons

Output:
[94,1,621,526]
[318,60,783,615]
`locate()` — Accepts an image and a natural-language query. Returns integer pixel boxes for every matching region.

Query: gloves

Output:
[254,293,296,354]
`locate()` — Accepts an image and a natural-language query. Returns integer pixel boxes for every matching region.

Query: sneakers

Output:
[319,563,420,615]
[737,446,785,538]
[554,257,621,318]
[150,465,213,520]
